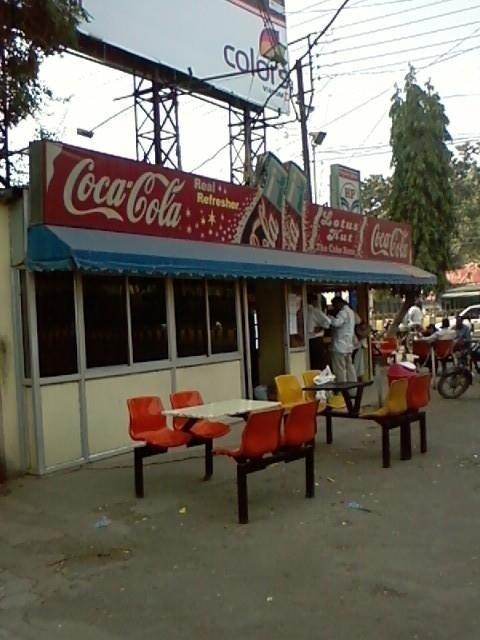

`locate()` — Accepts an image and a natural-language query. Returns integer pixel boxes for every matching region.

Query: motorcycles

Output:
[437,339,480,399]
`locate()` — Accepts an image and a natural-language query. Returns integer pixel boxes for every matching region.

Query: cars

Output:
[435,305,480,338]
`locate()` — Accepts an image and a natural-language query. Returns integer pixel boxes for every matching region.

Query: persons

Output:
[295,292,473,398]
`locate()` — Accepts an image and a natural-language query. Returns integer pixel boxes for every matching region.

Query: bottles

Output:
[239,157,307,252]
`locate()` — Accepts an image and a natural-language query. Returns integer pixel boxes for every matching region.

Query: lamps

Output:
[309,132,326,204]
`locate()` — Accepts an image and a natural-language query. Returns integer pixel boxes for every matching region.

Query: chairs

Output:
[126,383,324,526]
[271,369,435,471]
